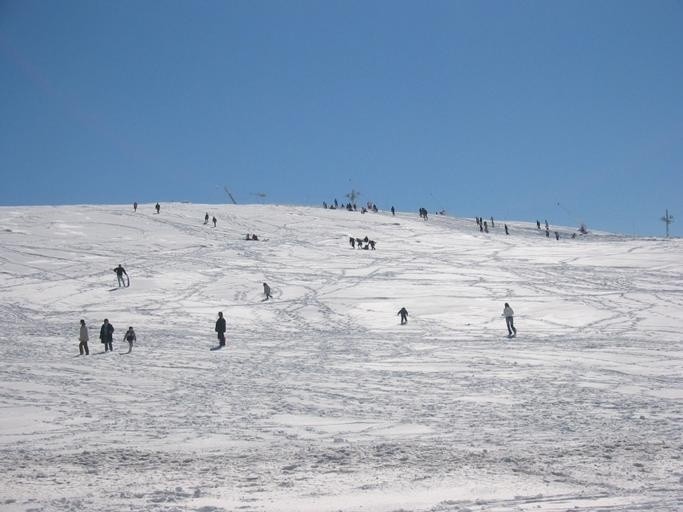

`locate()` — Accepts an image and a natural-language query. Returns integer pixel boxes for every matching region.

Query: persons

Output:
[122,326,136,354]
[78,319,88,355]
[474,214,494,233]
[418,207,427,219]
[348,235,376,251]
[262,282,272,300]
[202,212,208,224]
[503,224,509,235]
[214,312,225,346]
[133,202,137,211]
[390,206,394,215]
[397,308,408,324]
[212,217,216,227]
[500,303,516,337]
[320,197,378,213]
[98,319,114,352]
[245,233,257,240]
[155,203,159,214]
[536,219,575,241]
[112,264,127,288]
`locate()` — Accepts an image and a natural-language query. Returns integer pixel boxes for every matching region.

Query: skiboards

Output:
[108,286,130,291]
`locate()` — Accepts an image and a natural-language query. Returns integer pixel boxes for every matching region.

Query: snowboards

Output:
[261,297,269,302]
[73,354,90,357]
[93,349,117,355]
[120,352,131,355]
[210,346,224,349]
[400,322,408,324]
